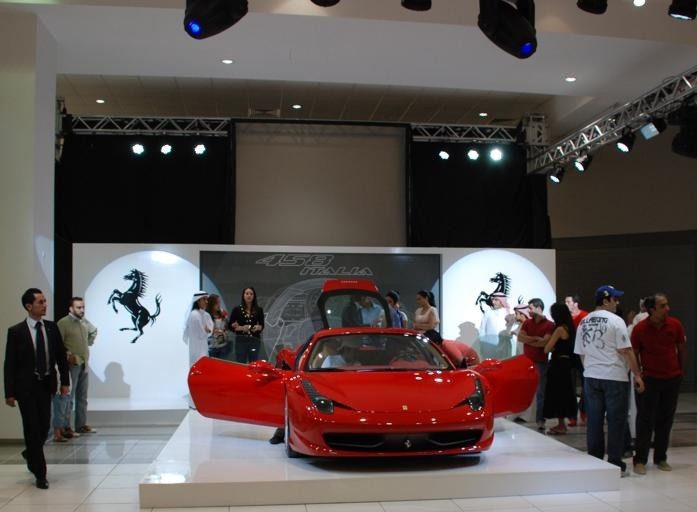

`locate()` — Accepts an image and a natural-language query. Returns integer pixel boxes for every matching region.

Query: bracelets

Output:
[318,353,324,359]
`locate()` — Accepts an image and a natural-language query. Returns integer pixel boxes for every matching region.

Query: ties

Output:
[35,321,47,379]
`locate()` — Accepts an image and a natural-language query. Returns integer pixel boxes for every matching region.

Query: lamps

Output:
[573,149,592,172]
[668,0,697,22]
[639,117,667,141]
[477,0,538,60]
[615,126,636,153]
[182,0,248,39]
[548,161,565,184]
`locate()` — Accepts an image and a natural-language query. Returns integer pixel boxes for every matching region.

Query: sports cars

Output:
[186,276,541,471]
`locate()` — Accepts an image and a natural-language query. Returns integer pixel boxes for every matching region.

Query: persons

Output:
[573,286,644,477]
[321,337,365,368]
[423,329,480,368]
[511,304,536,423]
[518,298,547,434]
[633,296,649,322]
[543,302,579,435]
[228,287,265,364]
[564,294,590,426]
[53,348,72,442]
[624,307,643,457]
[412,291,440,333]
[4,287,70,490]
[342,294,365,327]
[57,297,97,437]
[629,294,688,475]
[269,338,340,444]
[184,292,214,410]
[478,292,513,360]
[361,285,384,327]
[375,291,410,329]
[206,295,229,360]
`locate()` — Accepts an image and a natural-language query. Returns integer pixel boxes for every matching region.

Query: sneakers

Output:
[61,432,74,439]
[537,420,545,433]
[611,462,626,471]
[568,418,577,427]
[511,416,527,424]
[547,430,556,435]
[75,425,97,433]
[551,425,567,432]
[580,411,587,426]
[657,460,672,471]
[633,463,647,474]
[268,436,284,445]
[53,437,70,444]
[65,427,81,437]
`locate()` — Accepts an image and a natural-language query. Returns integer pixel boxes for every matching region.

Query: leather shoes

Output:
[36,477,49,489]
[21,449,35,475]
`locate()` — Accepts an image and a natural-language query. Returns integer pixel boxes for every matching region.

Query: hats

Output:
[594,285,624,299]
